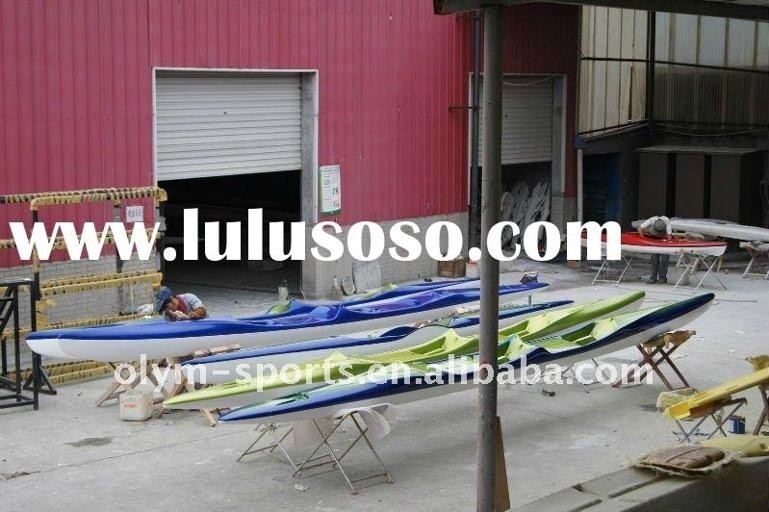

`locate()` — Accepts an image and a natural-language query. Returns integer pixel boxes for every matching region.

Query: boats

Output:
[564,227,728,256]
[630,216,769,243]
[27,274,716,427]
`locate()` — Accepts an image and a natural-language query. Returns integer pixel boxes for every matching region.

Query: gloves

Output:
[175,310,190,320]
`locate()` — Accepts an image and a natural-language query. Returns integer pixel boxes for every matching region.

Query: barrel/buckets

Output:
[666,266,690,286]
[277,278,289,303]
[118,389,154,421]
[727,415,747,433]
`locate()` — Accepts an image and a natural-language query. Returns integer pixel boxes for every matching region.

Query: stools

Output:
[237,402,393,495]
[95,343,240,428]
[549,330,692,395]
[591,242,769,295]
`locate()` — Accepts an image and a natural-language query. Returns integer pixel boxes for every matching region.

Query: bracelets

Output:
[666,238,669,241]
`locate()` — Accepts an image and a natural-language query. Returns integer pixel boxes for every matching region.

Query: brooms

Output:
[328,215,343,300]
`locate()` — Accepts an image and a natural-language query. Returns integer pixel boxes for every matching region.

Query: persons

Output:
[638,215,673,283]
[154,286,210,413]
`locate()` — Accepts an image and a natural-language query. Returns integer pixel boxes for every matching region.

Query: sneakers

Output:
[646,275,666,284]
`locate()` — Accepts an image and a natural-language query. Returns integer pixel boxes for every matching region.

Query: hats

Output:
[654,219,665,232]
[154,286,171,312]
